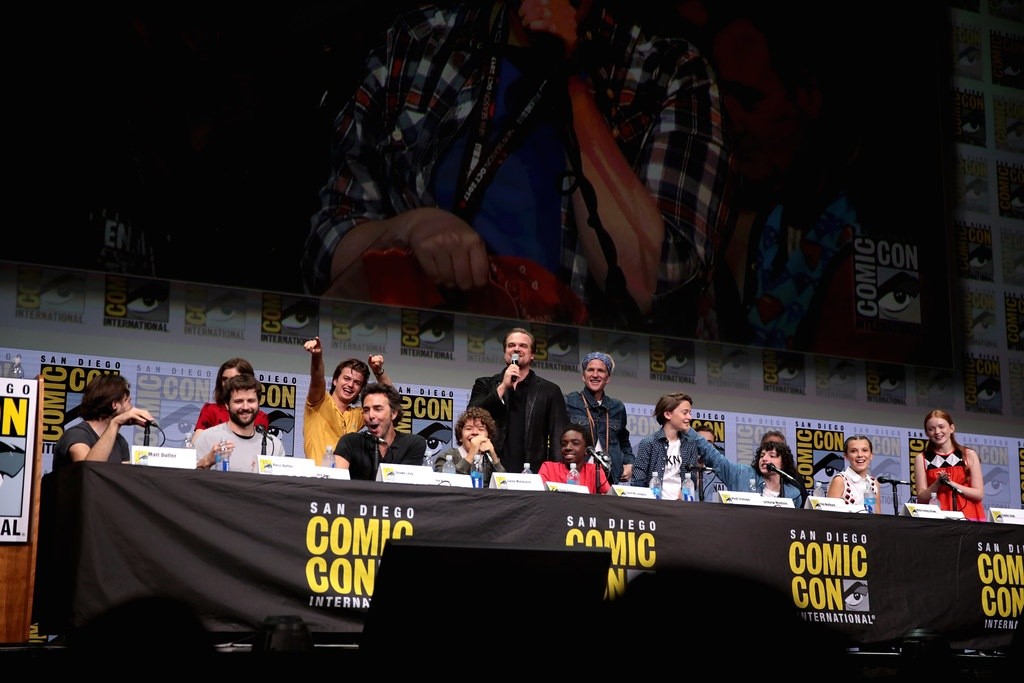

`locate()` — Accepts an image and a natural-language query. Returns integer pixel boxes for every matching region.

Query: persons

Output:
[630,391,802,509]
[288,0,725,337]
[564,351,635,486]
[826,435,881,514]
[53,373,157,471]
[194,372,285,473]
[302,335,395,467]
[332,382,426,482]
[915,409,987,521]
[538,423,610,495]
[434,406,506,487]
[467,328,566,474]
[192,358,268,449]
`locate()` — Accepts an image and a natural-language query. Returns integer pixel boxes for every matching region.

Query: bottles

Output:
[522,463,533,474]
[813,482,825,497]
[929,492,943,510]
[441,455,456,474]
[681,473,695,502]
[863,484,878,514]
[566,463,580,485]
[470,454,485,489]
[422,451,436,472]
[322,447,337,468]
[747,479,759,493]
[179,432,195,449]
[215,437,230,472]
[649,471,662,499]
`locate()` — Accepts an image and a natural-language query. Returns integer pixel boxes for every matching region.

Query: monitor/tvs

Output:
[358,538,613,653]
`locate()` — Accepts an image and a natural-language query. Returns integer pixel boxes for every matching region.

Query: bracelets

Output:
[375,368,384,378]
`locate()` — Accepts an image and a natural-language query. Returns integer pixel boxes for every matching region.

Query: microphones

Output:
[483,451,494,465]
[939,476,963,494]
[877,476,911,485]
[363,430,388,445]
[586,446,609,470]
[256,424,273,441]
[140,416,159,427]
[767,463,796,482]
[511,354,519,382]
[684,462,715,471]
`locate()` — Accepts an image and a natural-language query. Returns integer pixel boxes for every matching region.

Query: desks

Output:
[34,463,1024,650]
